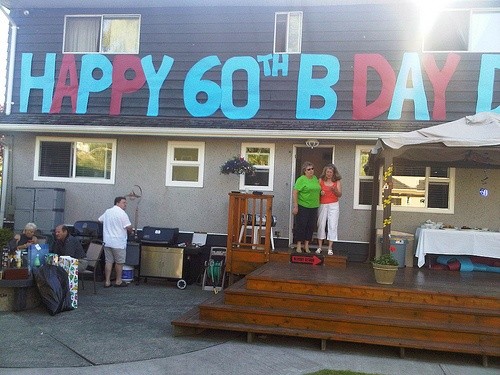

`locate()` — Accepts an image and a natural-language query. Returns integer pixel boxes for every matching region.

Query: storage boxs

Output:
[13,186,65,236]
[44,253,80,310]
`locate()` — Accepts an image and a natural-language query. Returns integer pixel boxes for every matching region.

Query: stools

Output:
[233,213,278,251]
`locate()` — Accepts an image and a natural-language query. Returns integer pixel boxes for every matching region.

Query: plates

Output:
[442,228,456,230]
[472,230,483,232]
[460,229,470,231]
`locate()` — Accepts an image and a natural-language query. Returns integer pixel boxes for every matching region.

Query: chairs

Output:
[79,239,106,295]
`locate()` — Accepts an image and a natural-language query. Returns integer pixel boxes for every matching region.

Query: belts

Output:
[81,256,87,259]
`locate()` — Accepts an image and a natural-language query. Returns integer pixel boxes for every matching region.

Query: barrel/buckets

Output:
[122,265,134,283]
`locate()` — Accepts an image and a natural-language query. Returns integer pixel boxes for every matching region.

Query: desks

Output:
[414,225,500,269]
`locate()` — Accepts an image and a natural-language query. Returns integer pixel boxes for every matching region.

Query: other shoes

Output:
[304,247,311,253]
[296,249,302,254]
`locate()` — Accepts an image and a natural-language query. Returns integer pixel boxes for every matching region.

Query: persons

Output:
[9,222,87,272]
[292,161,343,256]
[97,196,135,287]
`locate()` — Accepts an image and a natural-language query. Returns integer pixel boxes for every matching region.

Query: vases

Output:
[233,166,245,174]
[373,264,400,285]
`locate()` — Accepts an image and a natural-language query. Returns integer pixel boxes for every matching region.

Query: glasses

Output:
[307,168,314,171]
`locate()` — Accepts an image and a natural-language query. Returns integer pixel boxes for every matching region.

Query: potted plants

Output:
[448,258,460,270]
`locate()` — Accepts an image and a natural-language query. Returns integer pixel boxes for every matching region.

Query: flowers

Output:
[220,156,257,176]
[373,248,399,267]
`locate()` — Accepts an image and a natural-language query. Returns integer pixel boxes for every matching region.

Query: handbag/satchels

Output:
[31,263,75,316]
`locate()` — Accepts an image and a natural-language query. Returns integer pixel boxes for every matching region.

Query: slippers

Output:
[327,249,333,255]
[113,280,130,287]
[315,247,322,253]
[104,281,113,288]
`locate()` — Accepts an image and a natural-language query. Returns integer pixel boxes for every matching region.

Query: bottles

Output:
[34,254,40,267]
[9,249,28,269]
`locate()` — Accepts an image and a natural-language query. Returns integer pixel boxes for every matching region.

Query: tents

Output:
[365,111,500,266]
